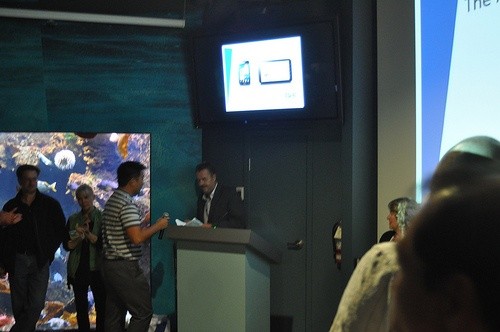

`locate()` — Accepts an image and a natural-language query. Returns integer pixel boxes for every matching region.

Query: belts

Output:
[104,257,139,262]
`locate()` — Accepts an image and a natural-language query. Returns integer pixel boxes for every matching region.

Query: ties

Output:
[206,199,209,217]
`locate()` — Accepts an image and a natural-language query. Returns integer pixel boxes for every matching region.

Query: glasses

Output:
[20,177,38,183]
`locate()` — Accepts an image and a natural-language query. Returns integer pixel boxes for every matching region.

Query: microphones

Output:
[214,201,231,229]
[158,212,169,239]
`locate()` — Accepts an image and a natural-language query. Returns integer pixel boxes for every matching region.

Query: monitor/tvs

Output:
[190,14,344,129]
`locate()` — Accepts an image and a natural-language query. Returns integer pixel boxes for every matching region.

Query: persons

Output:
[64,183,105,332]
[0,164,69,332]
[378,198,411,242]
[328,135,500,332]
[384,182,500,332]
[102,161,170,332]
[190,163,247,230]
